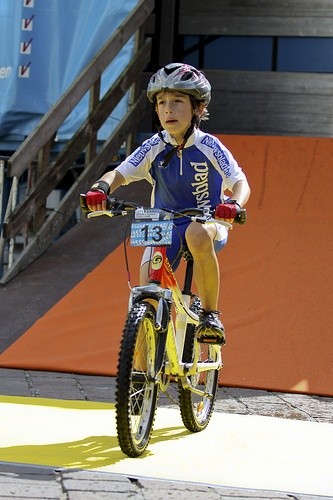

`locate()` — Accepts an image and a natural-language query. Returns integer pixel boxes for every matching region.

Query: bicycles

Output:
[81,193,246,457]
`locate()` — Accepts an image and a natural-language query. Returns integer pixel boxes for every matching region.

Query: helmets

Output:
[146,63,212,117]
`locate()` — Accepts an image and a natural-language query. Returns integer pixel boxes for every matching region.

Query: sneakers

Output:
[128,369,148,415]
[197,307,225,344]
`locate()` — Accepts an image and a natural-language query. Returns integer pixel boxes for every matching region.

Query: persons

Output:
[82,62,251,398]
